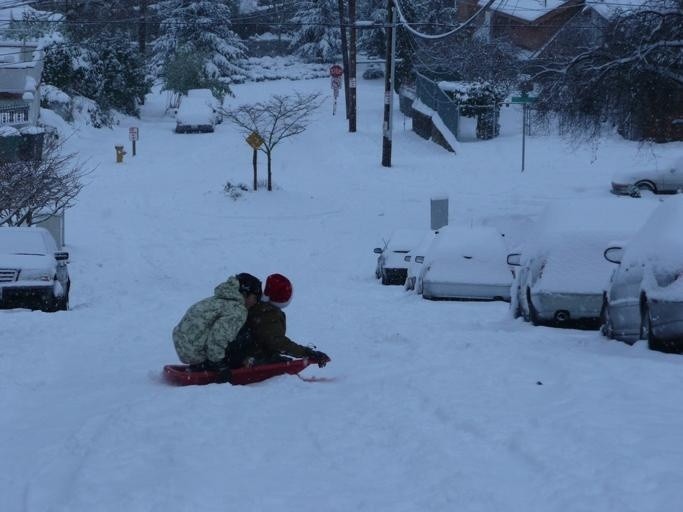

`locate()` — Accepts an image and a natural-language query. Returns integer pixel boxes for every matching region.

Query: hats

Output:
[263,274,293,306]
[238,273,262,298]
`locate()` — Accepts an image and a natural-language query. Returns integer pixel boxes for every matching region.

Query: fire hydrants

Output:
[114,145,127,162]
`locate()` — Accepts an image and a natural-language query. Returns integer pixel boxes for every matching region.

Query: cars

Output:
[174,88,224,134]
[0,225,72,314]
[609,150,683,198]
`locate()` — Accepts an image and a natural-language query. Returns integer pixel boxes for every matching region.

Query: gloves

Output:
[214,364,232,383]
[310,351,329,368]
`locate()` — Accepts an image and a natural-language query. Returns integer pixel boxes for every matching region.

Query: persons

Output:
[173,273,259,374]
[227,273,331,371]
